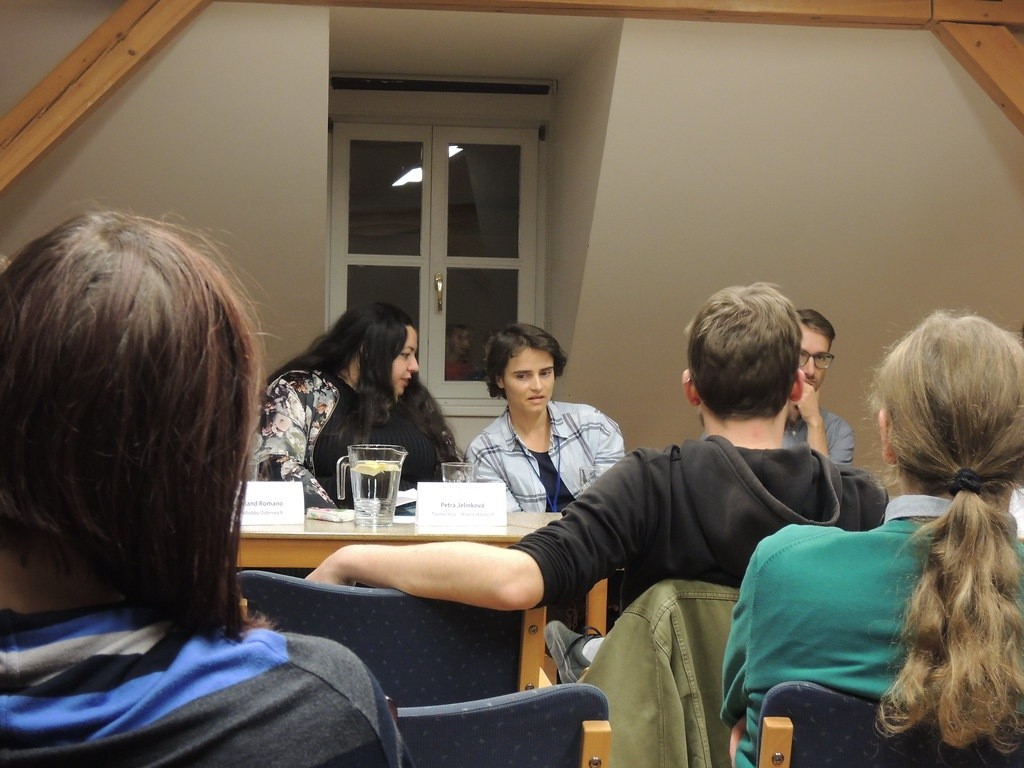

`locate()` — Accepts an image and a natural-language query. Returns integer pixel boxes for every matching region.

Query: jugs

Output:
[336,443,409,527]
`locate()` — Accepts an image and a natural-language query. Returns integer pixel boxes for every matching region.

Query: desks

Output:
[238,512,608,636]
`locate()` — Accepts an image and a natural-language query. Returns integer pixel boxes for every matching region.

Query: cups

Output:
[441,462,478,483]
[579,466,608,498]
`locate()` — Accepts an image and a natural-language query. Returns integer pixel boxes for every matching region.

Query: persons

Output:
[305,281,888,684]
[784,309,855,465]
[720,313,1024,767]
[446,324,474,380]
[248,302,461,511]
[473,328,497,379]
[0,210,406,768]
[463,324,625,514]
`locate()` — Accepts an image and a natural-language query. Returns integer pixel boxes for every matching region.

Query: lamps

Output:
[392,142,462,187]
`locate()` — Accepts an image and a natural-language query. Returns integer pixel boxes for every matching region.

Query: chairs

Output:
[756,681,1023,768]
[386,682,610,768]
[237,570,558,709]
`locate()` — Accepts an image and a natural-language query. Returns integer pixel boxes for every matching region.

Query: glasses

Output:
[799,348,835,369]
